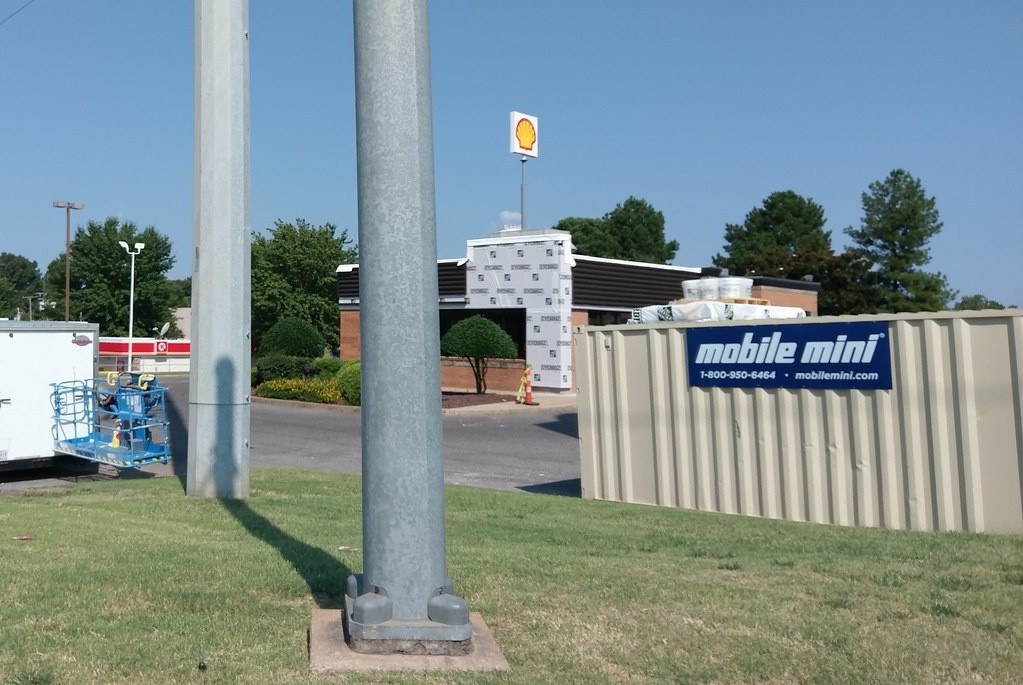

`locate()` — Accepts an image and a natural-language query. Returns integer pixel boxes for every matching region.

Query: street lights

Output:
[22,295,38,321]
[53,200,84,322]
[119,240,145,372]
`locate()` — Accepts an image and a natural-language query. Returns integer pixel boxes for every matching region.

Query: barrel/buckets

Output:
[680,277,754,300]
